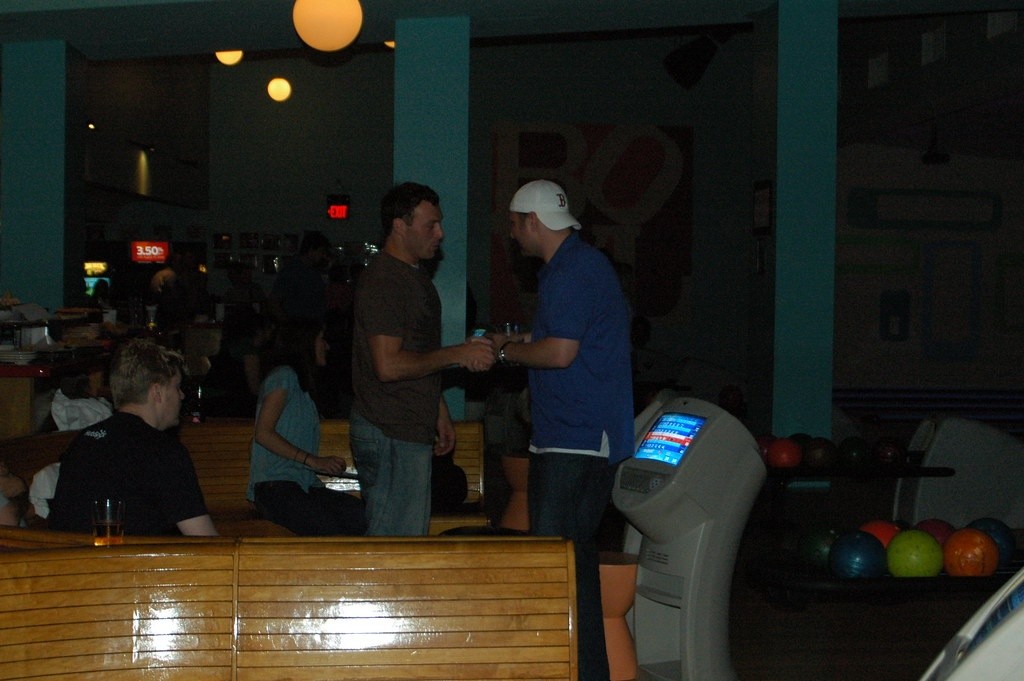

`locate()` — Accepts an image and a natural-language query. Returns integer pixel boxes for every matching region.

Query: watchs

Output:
[495,339,519,367]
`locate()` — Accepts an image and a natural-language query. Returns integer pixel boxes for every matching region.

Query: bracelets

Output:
[293,448,300,462]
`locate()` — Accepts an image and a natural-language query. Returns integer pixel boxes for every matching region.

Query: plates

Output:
[0,349,38,364]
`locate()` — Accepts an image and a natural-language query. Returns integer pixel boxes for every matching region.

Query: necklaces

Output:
[303,453,310,464]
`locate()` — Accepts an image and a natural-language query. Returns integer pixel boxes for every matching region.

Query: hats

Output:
[507,180,584,232]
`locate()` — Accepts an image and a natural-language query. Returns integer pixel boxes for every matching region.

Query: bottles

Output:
[189,385,206,424]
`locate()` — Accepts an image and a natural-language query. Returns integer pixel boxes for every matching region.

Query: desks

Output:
[0,355,109,437]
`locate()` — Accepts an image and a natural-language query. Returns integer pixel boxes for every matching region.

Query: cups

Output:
[88,496,127,539]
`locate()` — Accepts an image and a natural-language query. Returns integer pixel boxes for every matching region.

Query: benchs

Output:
[1,421,580,681]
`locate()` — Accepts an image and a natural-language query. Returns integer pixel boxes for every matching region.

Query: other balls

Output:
[754,430,910,469]
[795,517,1016,578]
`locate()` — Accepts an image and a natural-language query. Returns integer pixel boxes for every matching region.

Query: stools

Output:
[499,453,529,531]
[598,553,639,681]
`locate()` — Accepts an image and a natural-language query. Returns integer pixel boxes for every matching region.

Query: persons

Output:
[246,317,366,537]
[0,449,47,530]
[460,179,634,681]
[46,328,219,537]
[645,372,681,403]
[37,235,350,419]
[350,182,494,537]
